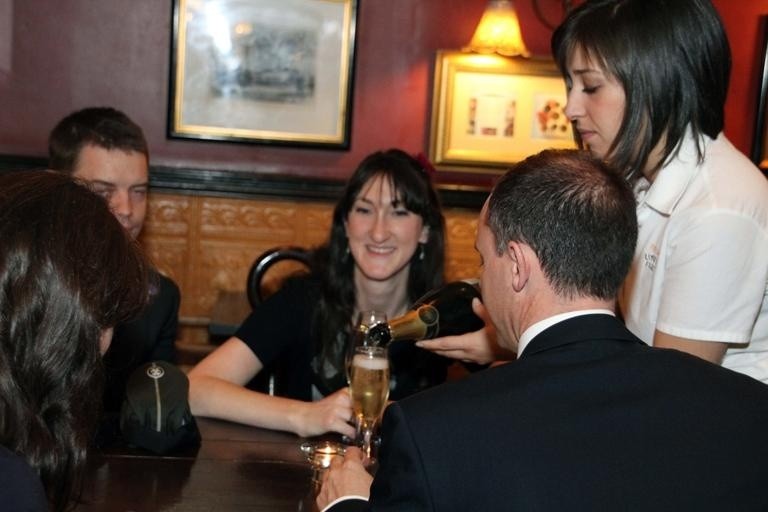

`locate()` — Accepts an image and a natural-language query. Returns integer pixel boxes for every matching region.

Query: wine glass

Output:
[347,348,389,465]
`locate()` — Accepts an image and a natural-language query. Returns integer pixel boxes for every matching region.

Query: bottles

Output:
[364,277,483,347]
[467,98,477,133]
[502,98,518,137]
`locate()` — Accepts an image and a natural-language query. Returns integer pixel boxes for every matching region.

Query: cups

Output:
[352,309,389,346]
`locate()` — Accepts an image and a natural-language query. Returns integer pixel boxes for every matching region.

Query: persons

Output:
[44,107,181,472]
[1,169,159,512]
[186,146,454,438]
[315,147,768,512]
[416,0,768,385]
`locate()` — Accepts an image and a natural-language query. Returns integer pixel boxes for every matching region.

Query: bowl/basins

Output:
[295,438,348,467]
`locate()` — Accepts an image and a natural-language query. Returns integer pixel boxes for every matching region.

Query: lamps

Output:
[459,0,575,61]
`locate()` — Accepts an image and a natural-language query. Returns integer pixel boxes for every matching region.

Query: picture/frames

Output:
[166,0,359,151]
[427,47,590,174]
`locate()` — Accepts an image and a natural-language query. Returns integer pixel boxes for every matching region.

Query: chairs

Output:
[242,247,320,398]
